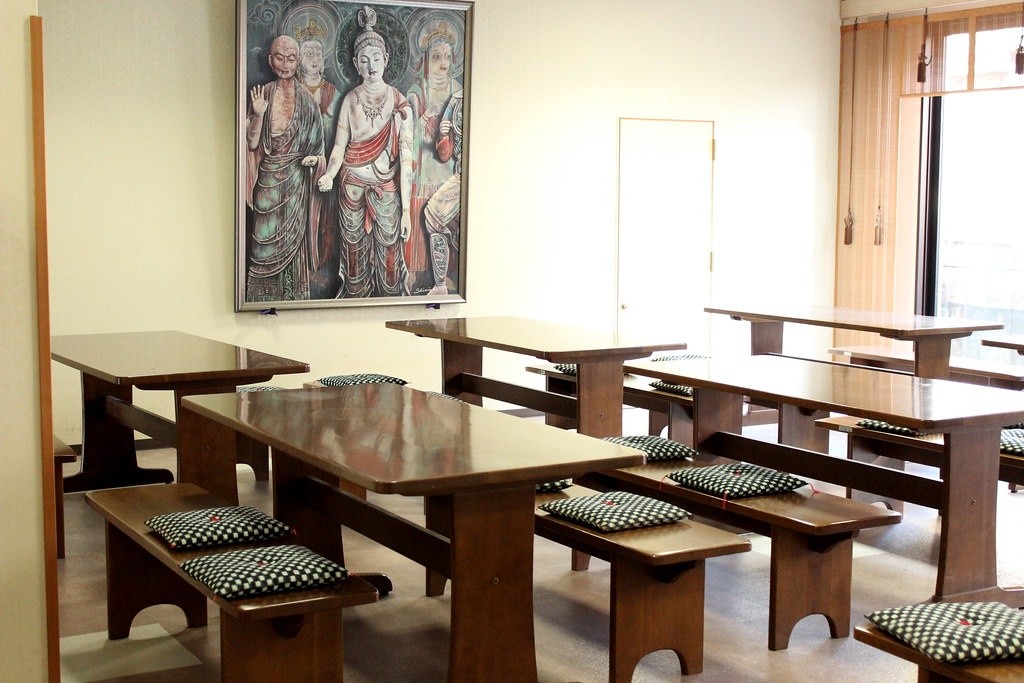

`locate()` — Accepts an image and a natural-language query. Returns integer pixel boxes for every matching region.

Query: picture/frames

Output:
[233,0,475,313]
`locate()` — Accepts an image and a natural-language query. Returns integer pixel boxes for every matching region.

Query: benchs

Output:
[52,345,1024,683]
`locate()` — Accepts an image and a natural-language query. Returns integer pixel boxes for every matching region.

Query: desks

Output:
[384,315,687,443]
[634,355,1024,593]
[982,336,1024,354]
[703,304,1005,379]
[49,331,310,506]
[181,384,644,683]
